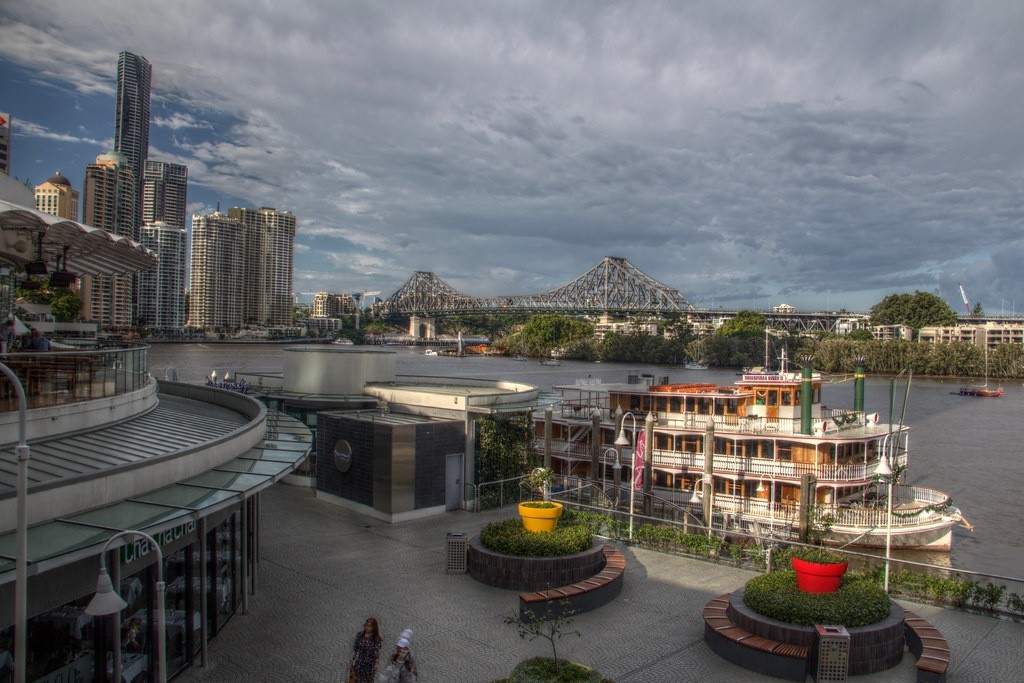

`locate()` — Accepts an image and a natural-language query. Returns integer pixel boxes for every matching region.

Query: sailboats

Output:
[734,325,781,376]
[682,331,710,370]
[959,340,1005,398]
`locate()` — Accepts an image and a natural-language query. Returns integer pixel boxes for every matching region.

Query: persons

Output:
[22,328,52,374]
[349,617,383,683]
[121,617,147,653]
[0,319,16,360]
[45,646,76,683]
[378,639,418,683]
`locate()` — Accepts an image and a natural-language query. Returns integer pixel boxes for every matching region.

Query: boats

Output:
[332,336,355,346]
[425,349,438,356]
[512,348,976,553]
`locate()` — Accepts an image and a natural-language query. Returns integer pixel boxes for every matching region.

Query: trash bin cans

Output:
[812,625,850,683]
[446,532,467,574]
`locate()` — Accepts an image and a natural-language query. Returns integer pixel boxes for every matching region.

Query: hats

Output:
[396,639,409,647]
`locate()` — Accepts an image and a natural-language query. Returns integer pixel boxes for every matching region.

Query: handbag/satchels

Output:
[349,662,356,683]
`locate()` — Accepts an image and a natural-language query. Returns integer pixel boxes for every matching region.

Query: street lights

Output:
[613,412,636,540]
[756,473,774,547]
[871,433,895,595]
[689,478,714,545]
[83,530,168,683]
[603,448,623,508]
